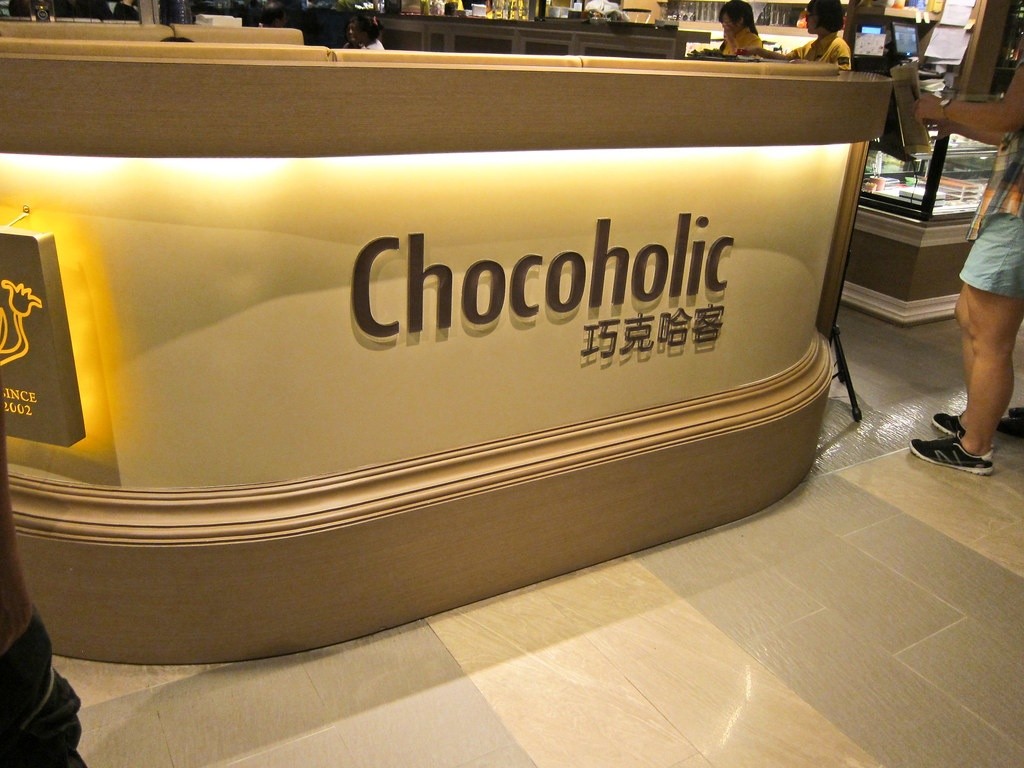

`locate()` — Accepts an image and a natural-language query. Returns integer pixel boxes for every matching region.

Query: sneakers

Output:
[909,431,994,476]
[932,413,967,436]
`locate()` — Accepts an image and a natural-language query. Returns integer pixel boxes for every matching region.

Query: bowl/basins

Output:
[905,176,916,182]
[906,182,915,186]
[622,8,652,24]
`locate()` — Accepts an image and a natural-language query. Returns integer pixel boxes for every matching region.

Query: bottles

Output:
[871,0,906,8]
[27,0,56,22]
[491,0,528,20]
[176,0,192,24]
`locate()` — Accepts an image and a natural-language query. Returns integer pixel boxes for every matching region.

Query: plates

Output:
[700,54,734,61]
[886,178,900,187]
[737,54,789,63]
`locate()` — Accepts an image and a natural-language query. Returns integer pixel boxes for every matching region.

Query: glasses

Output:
[807,11,818,18]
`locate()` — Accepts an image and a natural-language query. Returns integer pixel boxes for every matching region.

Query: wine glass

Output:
[679,1,695,22]
[666,9,678,22]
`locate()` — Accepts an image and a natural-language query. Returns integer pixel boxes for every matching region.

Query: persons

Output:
[911,57,1024,476]
[719,0,763,56]
[753,0,852,71]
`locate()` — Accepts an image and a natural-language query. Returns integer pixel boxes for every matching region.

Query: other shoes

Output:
[997,417,1024,437]
[1008,408,1024,419]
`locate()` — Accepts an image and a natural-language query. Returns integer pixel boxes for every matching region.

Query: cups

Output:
[869,176,885,191]
[574,3,582,12]
[696,2,724,22]
[548,6,560,18]
[758,3,793,26]
[864,186,876,191]
[560,6,569,18]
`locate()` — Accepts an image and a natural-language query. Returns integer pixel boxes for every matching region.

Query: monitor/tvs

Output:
[891,21,919,57]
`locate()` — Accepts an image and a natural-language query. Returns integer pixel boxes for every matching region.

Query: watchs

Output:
[939,98,953,120]
